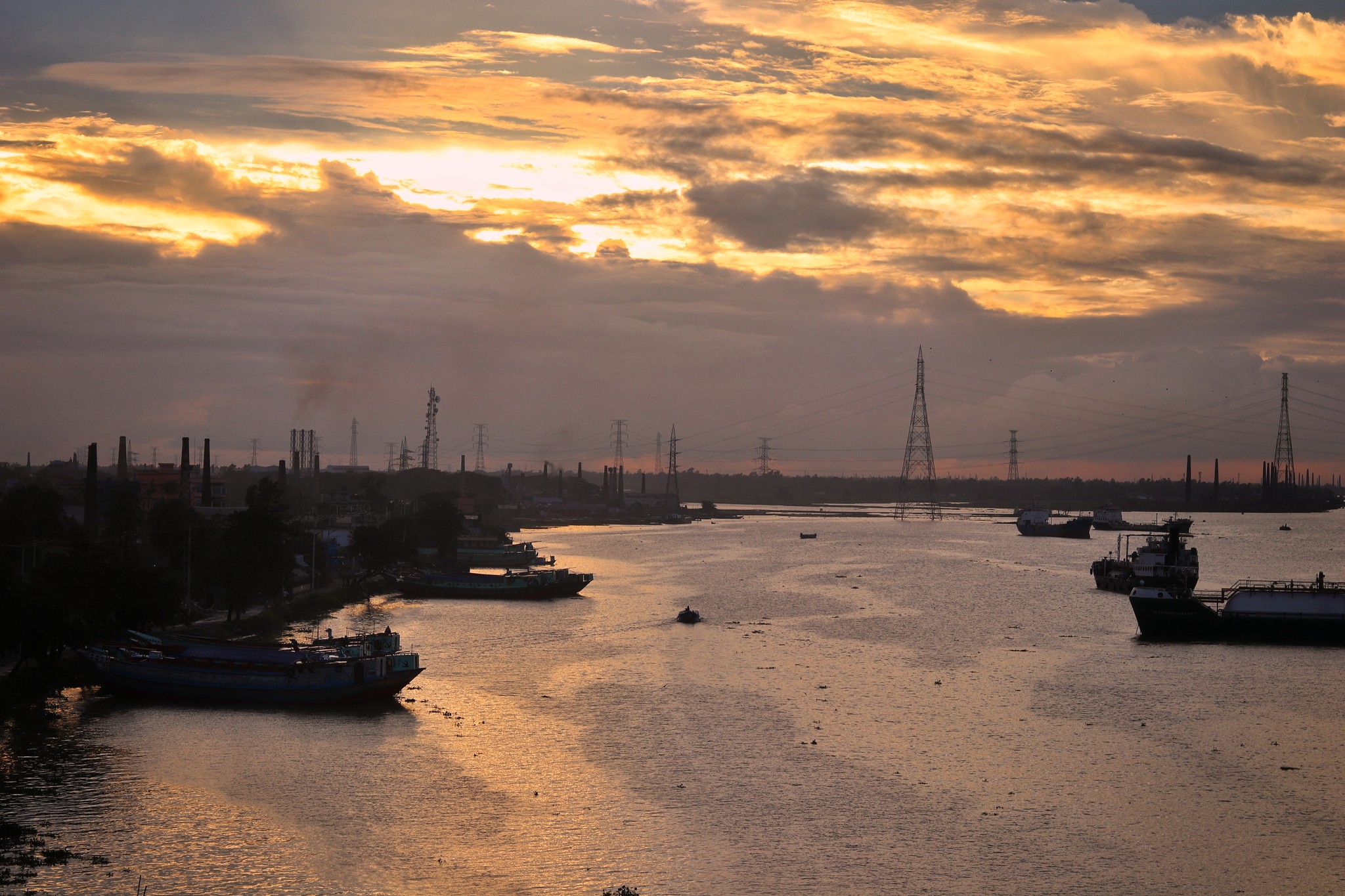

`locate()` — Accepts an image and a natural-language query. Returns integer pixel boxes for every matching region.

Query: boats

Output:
[1016,508,1092,539]
[800,532,817,538]
[677,609,701,624]
[527,555,556,565]
[418,537,539,567]
[1279,527,1291,531]
[64,620,427,717]
[380,550,594,603]
[1092,504,1194,534]
[1090,522,1200,598]
[1129,570,1345,648]
